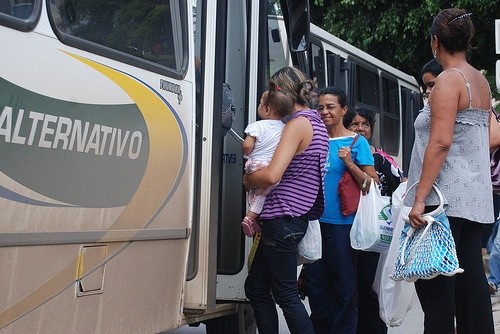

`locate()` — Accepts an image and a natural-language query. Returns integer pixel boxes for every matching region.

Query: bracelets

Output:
[362,172,367,188]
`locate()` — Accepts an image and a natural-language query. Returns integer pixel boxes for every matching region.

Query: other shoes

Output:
[488,281,497,294]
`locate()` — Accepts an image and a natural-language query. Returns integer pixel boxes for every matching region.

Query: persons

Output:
[244,67,329,334]
[305,86,379,334]
[242,90,287,237]
[344,106,400,334]
[404,7,500,334]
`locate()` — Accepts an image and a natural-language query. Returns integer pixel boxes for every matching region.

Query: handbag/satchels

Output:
[338,168,361,217]
[388,180,464,283]
[349,176,393,253]
[297,220,322,265]
[372,179,414,328]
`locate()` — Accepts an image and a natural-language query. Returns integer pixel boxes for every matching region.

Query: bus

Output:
[0,0,427,334]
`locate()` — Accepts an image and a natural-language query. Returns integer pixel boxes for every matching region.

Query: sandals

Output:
[242,216,255,237]
